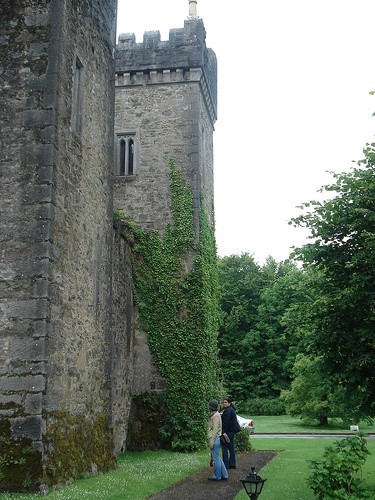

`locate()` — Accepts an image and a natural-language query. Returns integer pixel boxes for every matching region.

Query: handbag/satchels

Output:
[220,433,231,444]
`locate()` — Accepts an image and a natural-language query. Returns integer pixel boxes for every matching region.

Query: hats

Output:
[208,399,218,410]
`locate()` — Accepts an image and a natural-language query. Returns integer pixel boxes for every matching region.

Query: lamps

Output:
[240,467,267,500]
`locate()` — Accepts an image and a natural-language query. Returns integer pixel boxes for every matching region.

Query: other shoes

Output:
[221,477,228,480]
[229,465,234,468]
[208,476,215,479]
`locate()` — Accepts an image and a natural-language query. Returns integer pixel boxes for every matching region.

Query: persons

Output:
[220,399,241,469]
[207,399,229,481]
